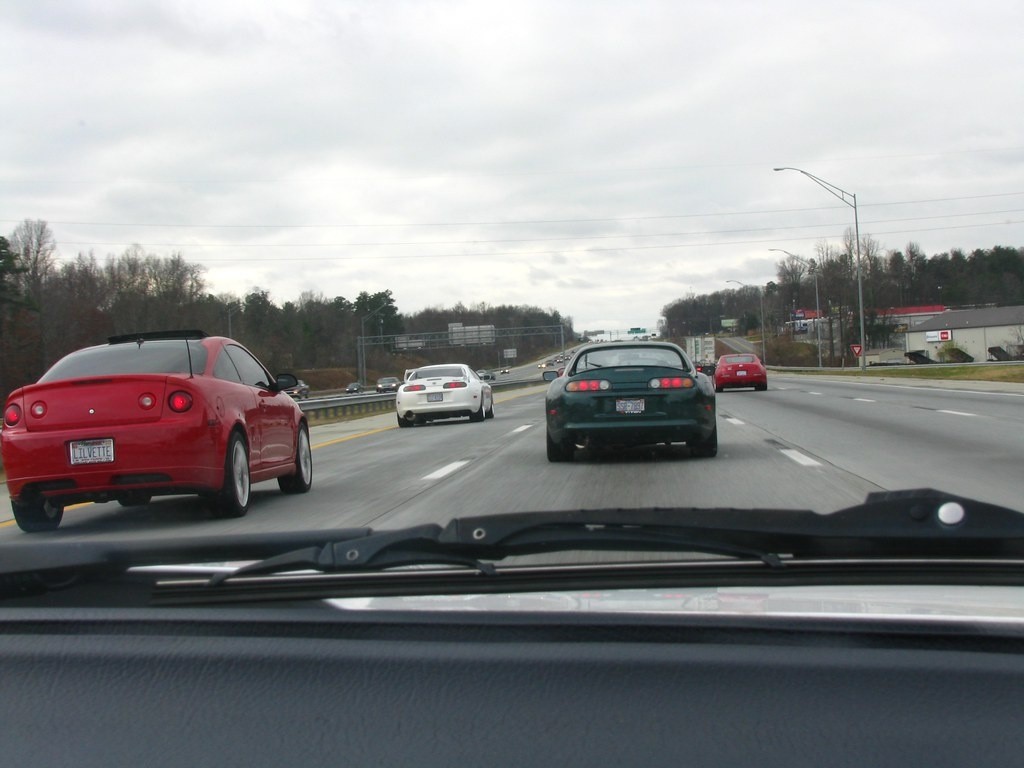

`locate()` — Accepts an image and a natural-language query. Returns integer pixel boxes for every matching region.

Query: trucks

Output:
[685,335,718,376]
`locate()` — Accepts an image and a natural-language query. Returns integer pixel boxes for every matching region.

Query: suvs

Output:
[283,379,310,399]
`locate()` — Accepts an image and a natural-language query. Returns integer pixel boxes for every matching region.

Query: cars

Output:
[538,349,577,368]
[712,353,769,393]
[541,341,717,463]
[376,377,404,394]
[394,362,495,428]
[0,327,315,535]
[476,369,496,381]
[500,368,509,374]
[345,382,365,393]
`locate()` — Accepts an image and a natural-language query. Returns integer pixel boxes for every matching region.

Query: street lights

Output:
[769,248,822,367]
[773,166,866,371]
[361,302,387,386]
[726,279,766,365]
